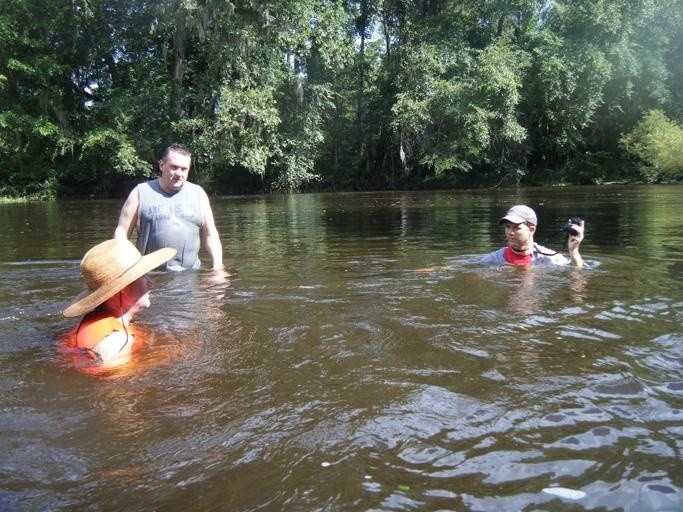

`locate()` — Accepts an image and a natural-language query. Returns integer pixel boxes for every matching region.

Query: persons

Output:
[475,205,585,269]
[61,237,178,382]
[112,143,226,287]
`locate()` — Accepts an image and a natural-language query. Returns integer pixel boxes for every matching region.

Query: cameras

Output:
[560,216,579,235]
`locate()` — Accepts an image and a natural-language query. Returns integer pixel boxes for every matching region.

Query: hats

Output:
[58,233,178,319]
[495,204,539,229]
